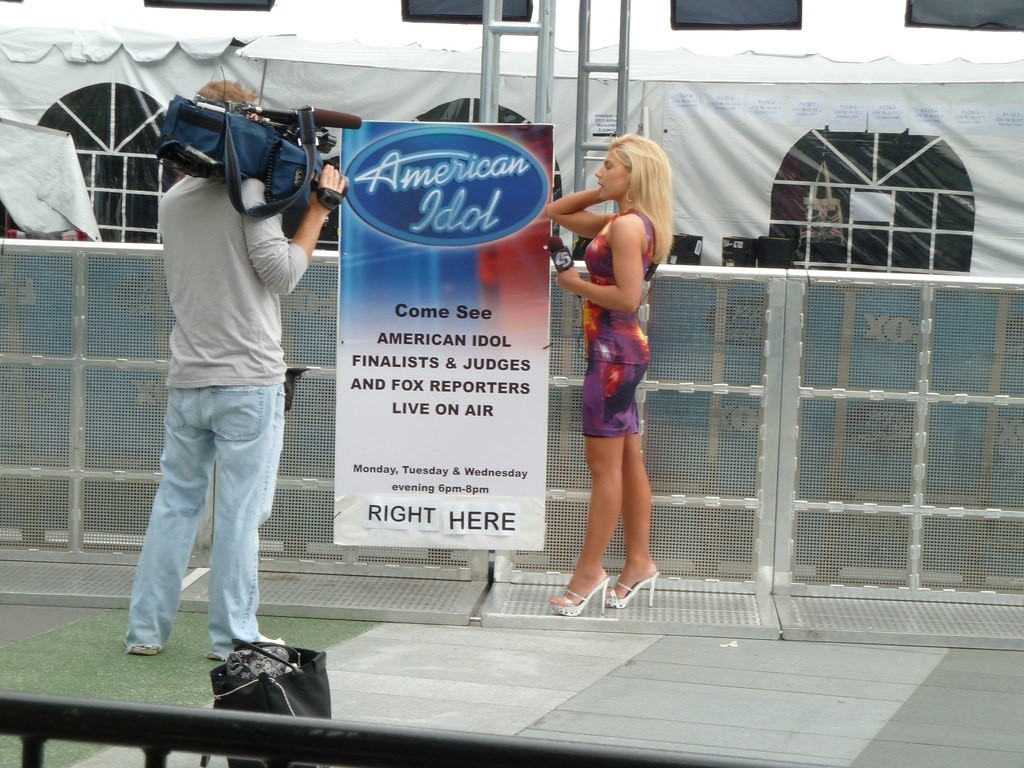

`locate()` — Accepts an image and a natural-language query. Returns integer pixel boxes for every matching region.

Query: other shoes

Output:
[124,628,158,655]
[208,634,284,660]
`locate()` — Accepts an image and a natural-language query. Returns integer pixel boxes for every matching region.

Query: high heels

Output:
[604,571,661,608]
[548,577,610,616]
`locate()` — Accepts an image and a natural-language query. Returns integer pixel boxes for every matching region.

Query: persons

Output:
[546,135,674,617]
[121,79,347,660]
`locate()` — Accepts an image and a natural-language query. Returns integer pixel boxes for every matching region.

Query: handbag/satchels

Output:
[208,639,331,768]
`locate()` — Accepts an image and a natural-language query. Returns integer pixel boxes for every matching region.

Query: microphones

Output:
[254,107,363,130]
[548,235,582,298]
